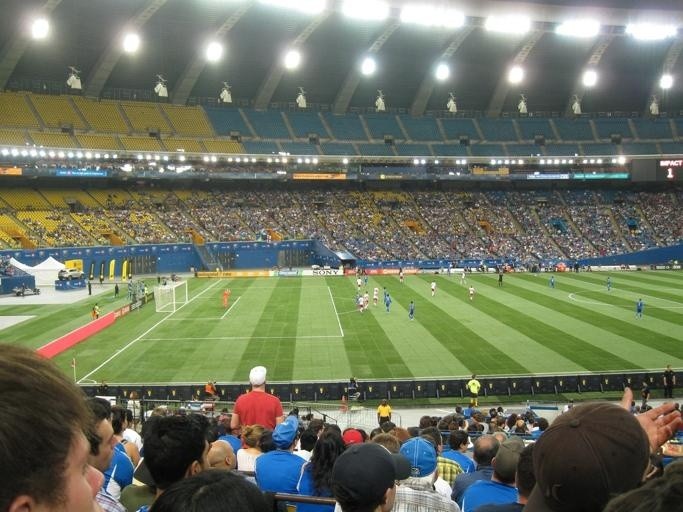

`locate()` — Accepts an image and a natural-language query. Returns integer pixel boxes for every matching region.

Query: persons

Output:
[82,365,682,512]
[0,344,103,512]
[3,189,682,320]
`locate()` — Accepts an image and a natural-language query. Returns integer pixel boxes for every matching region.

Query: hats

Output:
[494,401,650,512]
[343,430,364,446]
[398,438,437,477]
[272,416,299,449]
[249,366,267,386]
[331,442,411,505]
